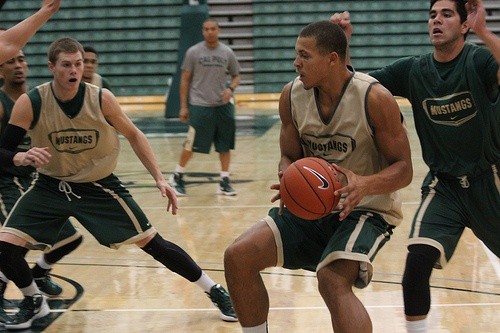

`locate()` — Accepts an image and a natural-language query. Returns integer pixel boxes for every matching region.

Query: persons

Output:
[327,0,500,333]
[168,20,241,197]
[222,19,413,333]
[0,38,241,328]
[0,0,61,67]
[0,49,82,314]
[80,45,110,95]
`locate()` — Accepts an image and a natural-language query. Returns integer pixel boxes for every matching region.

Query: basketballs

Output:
[279,157,342,219]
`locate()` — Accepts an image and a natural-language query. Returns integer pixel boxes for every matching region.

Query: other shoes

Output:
[167,172,186,196]
[218,175,238,195]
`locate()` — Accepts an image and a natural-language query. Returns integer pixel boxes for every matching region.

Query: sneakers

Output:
[32,273,62,298]
[204,283,238,322]
[3,294,51,329]
[0,306,9,326]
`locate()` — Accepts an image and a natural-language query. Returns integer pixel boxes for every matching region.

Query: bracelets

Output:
[228,86,235,92]
